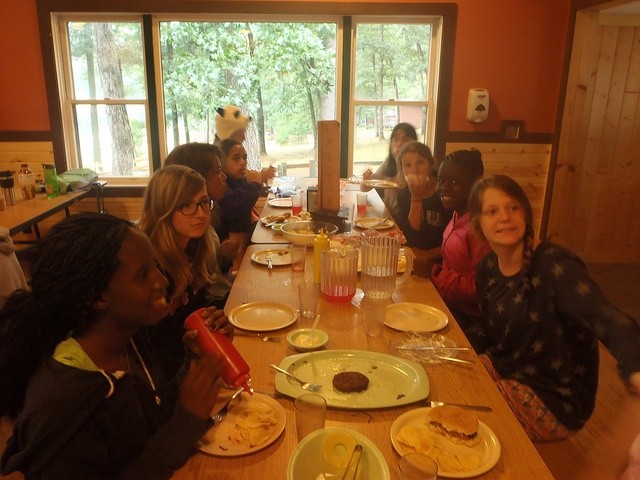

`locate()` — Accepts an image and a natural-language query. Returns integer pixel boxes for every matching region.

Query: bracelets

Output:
[409,198,423,203]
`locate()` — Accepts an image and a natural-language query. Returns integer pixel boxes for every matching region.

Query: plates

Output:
[268,198,293,207]
[229,301,299,331]
[389,407,501,478]
[276,349,429,411]
[357,217,396,231]
[381,301,450,335]
[250,247,297,266]
[196,392,285,458]
[363,181,400,190]
[261,215,301,226]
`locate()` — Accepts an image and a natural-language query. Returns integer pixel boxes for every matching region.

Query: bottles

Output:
[43,164,59,200]
[314,227,329,286]
[184,306,254,396]
[17,163,36,200]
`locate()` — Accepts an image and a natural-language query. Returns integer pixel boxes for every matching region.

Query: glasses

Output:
[174,197,214,216]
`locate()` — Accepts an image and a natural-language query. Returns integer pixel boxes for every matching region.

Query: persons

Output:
[213,129,277,183]
[162,142,251,245]
[215,139,262,230]
[392,142,453,259]
[1,213,233,480]
[412,148,493,305]
[463,173,640,443]
[138,165,243,350]
[361,124,417,215]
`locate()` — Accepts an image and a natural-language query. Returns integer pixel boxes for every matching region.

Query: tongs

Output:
[338,444,363,480]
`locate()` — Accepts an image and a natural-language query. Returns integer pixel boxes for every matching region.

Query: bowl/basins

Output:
[281,221,339,247]
[287,429,390,479]
[287,329,328,351]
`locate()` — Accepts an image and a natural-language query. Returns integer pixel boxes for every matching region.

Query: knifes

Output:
[265,213,289,227]
[414,400,495,411]
[394,345,471,351]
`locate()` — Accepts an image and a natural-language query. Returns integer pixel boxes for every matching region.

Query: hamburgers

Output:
[426,405,482,447]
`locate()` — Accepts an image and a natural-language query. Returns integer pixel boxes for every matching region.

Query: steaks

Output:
[333,373,368,391]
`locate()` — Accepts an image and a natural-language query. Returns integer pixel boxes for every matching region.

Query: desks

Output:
[0,179,109,256]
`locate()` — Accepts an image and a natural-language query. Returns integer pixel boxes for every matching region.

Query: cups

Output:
[291,196,302,216]
[398,454,438,480]
[362,237,414,300]
[298,281,321,319]
[294,395,326,444]
[363,297,383,339]
[356,193,367,216]
[291,243,306,273]
[321,250,357,306]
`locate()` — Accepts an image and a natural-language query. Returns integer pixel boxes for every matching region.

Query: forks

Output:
[266,257,273,269]
[212,379,254,422]
[413,349,475,366]
[268,364,321,392]
[415,399,493,412]
